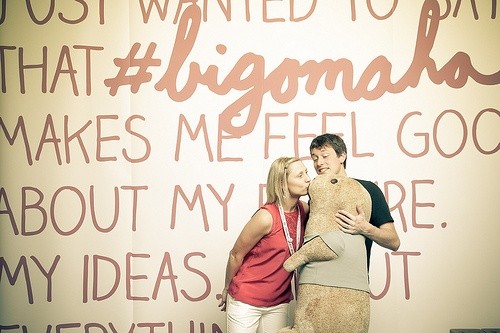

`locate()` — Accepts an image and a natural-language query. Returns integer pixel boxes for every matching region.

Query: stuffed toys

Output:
[281,175,372,333]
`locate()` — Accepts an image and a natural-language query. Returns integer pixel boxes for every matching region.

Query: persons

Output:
[219,158,311,333]
[306,134,400,284]
